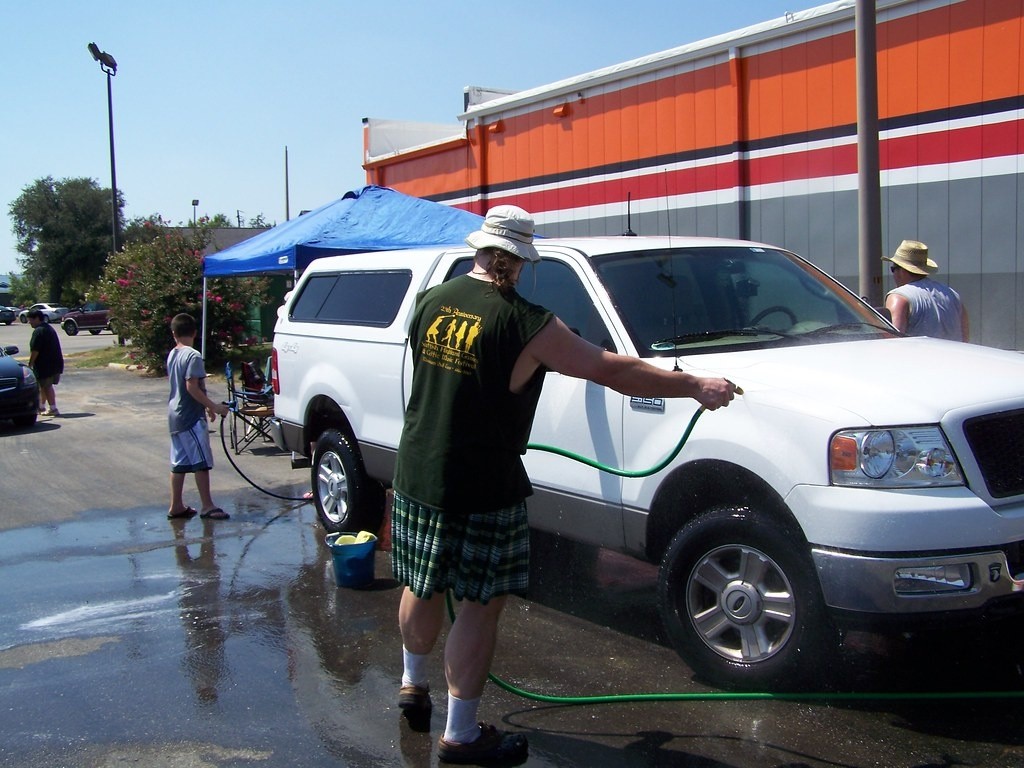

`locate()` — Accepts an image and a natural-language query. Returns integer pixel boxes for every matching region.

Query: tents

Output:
[205,184,551,368]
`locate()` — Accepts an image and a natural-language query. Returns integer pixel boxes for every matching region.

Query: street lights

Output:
[88,42,120,254]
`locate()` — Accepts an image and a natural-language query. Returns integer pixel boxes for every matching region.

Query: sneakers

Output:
[399,684,430,706]
[37,408,46,415]
[44,408,60,415]
[437,721,525,759]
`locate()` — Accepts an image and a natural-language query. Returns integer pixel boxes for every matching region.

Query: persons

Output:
[26,310,64,416]
[167,314,230,519]
[392,205,744,763]
[881,239,969,342]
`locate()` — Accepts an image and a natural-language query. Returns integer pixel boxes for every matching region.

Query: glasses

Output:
[890,265,900,272]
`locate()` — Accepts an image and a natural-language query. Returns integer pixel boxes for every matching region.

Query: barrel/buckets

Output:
[324,532,378,589]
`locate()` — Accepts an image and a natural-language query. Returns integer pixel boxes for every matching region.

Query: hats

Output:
[465,204,540,262]
[880,239,938,275]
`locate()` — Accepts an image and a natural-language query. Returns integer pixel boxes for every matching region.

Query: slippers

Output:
[167,505,197,517]
[200,507,230,519]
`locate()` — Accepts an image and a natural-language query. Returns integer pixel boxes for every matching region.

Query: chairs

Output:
[226,356,275,456]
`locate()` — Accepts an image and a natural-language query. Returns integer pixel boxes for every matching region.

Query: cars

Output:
[0,345,40,426]
[0,306,17,325]
[18,302,70,324]
[0,281,14,294]
[5,307,22,317]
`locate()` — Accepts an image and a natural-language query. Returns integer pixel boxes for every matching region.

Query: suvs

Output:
[60,298,121,336]
[267,235,1023,695]
